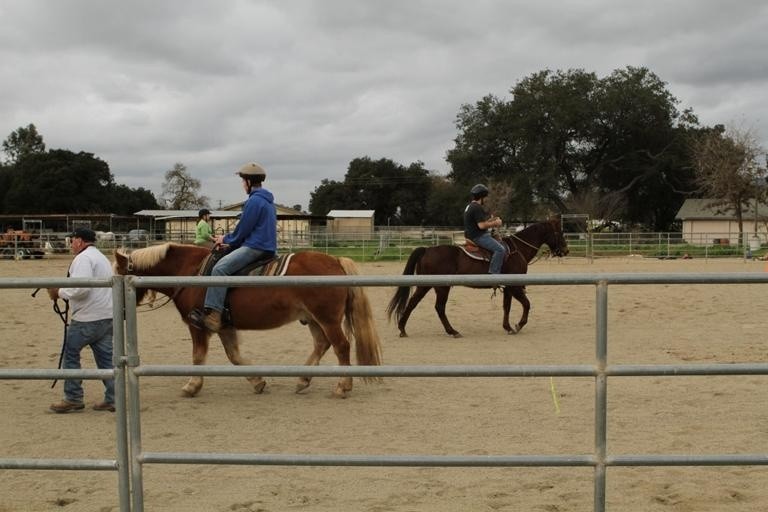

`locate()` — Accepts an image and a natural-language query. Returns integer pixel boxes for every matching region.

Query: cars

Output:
[121,229,150,247]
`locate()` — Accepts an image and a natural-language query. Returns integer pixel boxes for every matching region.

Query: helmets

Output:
[198,209,212,216]
[235,163,265,182]
[470,184,489,194]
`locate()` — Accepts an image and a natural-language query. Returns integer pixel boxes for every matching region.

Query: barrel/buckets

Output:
[749,235,761,251]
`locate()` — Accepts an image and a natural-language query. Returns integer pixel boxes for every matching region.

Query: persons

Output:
[193,209,217,246]
[189,162,277,332]
[464,183,506,275]
[49,228,121,413]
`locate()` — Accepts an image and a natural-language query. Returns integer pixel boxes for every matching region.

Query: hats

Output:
[64,227,96,242]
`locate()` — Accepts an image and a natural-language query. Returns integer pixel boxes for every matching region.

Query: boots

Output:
[188,306,222,333]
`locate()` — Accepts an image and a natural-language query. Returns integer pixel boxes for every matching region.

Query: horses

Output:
[112,241,385,400]
[385,213,570,339]
[95,230,115,248]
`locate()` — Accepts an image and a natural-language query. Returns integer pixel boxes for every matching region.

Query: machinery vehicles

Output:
[0,218,45,259]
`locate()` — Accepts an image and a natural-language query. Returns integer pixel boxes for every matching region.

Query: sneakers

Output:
[92,401,116,413]
[48,400,85,413]
